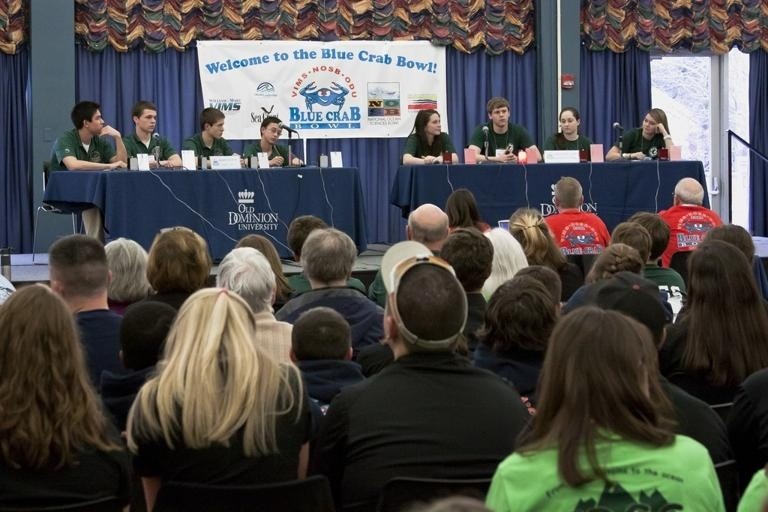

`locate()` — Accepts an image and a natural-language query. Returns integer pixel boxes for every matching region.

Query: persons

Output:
[538,108,594,162]
[44,100,127,243]
[400,108,460,163]
[175,105,235,166]
[111,100,183,166]
[1,172,767,509]
[602,107,688,161]
[467,97,543,160]
[237,116,303,167]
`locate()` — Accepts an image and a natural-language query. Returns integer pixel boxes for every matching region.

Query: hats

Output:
[593,268,666,346]
[378,237,471,354]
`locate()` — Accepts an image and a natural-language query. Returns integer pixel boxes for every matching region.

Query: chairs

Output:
[32,160,85,265]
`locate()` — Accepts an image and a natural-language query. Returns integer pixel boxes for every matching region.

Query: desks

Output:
[41,166,368,261]
[388,161,711,236]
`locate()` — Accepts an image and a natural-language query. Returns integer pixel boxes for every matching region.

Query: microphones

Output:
[482,126,489,147]
[612,122,624,132]
[278,121,297,134]
[153,131,161,143]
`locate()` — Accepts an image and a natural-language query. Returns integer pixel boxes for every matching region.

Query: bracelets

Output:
[663,135,672,140]
